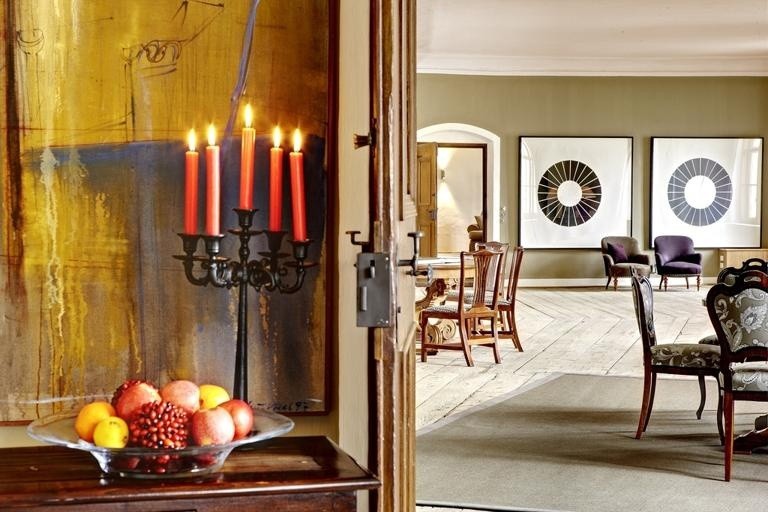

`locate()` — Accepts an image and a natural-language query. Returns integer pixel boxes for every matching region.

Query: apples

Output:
[191,406,235,446]
[159,380,202,417]
[117,383,162,418]
[215,400,254,440]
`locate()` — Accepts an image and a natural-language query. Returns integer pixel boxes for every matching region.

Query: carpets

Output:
[415,370,768,512]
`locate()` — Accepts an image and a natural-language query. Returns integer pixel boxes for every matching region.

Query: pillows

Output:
[473,215,484,230]
[608,244,630,264]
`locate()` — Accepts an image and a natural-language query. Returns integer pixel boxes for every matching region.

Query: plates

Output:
[24,406,295,483]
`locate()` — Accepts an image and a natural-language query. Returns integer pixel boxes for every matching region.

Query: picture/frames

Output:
[649,135,764,250]
[517,136,633,250]
[0,1,342,427]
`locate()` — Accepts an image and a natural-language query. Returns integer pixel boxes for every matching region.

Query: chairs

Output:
[420,240,524,368]
[698,258,767,348]
[705,267,768,482]
[601,235,651,291]
[630,264,725,441]
[655,235,700,292]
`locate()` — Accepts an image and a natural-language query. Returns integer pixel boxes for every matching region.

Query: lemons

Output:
[198,384,230,411]
[94,416,129,447]
[74,400,116,443]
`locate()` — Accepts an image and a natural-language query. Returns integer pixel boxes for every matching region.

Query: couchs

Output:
[468,212,483,251]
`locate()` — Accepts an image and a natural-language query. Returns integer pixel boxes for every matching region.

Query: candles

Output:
[237,102,256,211]
[269,125,283,232]
[183,126,199,233]
[205,121,220,233]
[287,127,309,241]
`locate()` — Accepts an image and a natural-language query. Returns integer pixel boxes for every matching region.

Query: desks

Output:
[0,436,381,512]
[719,249,768,271]
